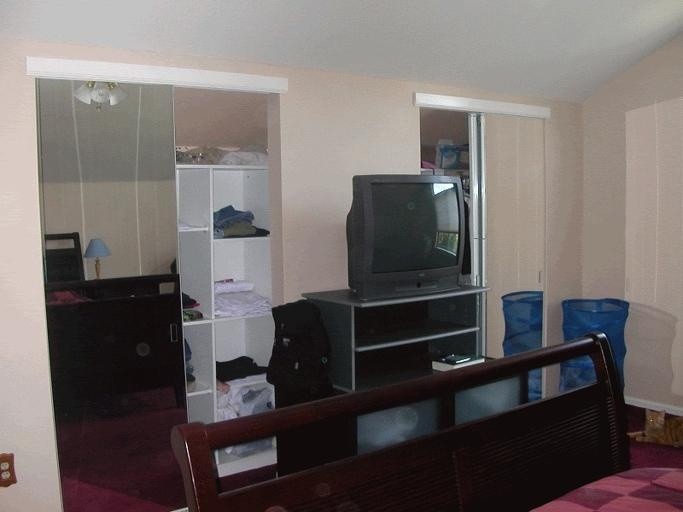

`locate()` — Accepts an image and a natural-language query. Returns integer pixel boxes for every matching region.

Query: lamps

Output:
[73,82,127,112]
[84,238,111,280]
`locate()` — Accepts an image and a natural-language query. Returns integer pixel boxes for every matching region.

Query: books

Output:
[431,352,485,372]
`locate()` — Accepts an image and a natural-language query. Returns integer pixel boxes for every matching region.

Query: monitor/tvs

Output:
[345,174,466,302]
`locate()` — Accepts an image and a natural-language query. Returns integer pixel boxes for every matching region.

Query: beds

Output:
[172,333,682,512]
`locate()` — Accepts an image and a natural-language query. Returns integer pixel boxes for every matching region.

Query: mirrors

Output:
[35,76,191,512]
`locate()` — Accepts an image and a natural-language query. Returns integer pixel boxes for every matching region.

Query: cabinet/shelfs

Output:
[302,286,491,394]
[176,165,279,476]
[44,273,182,399]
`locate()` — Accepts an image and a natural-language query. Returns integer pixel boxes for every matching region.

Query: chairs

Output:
[45,231,85,283]
